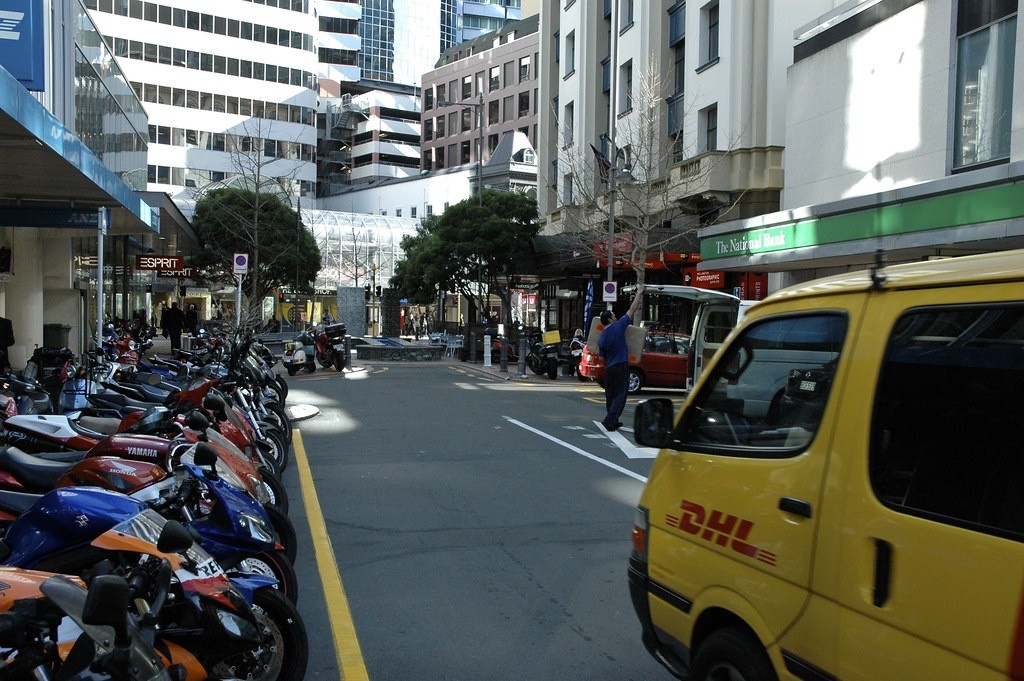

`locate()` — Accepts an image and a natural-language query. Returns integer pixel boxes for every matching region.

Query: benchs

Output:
[258,332,299,344]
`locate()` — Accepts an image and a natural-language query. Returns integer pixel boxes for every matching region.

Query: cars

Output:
[578,328,691,395]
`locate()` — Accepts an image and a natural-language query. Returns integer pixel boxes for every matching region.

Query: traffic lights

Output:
[365,283,370,301]
[376,285,381,296]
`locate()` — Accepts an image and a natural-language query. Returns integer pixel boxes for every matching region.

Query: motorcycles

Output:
[0,317,310,681]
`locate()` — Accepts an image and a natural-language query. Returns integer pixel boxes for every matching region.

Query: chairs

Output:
[152,94,164,104]
[182,98,198,108]
[240,108,252,116]
[270,110,279,120]
[208,101,225,112]
[428,332,464,358]
[255,106,266,118]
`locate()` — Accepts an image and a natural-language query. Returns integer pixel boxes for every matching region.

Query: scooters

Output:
[281,317,349,376]
[525,328,561,380]
[485,326,535,363]
[569,338,589,382]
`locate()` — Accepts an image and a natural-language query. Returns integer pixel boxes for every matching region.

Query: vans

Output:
[625,247,1024,681]
[622,284,847,420]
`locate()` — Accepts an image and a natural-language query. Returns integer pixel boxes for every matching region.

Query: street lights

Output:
[437,93,483,323]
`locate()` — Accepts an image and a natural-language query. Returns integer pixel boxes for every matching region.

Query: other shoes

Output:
[601,419,623,431]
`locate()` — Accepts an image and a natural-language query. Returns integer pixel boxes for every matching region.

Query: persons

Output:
[460,314,464,326]
[323,309,333,325]
[598,284,646,431]
[161,302,185,354]
[0,317,16,374]
[404,311,435,341]
[185,305,198,331]
[514,317,520,324]
